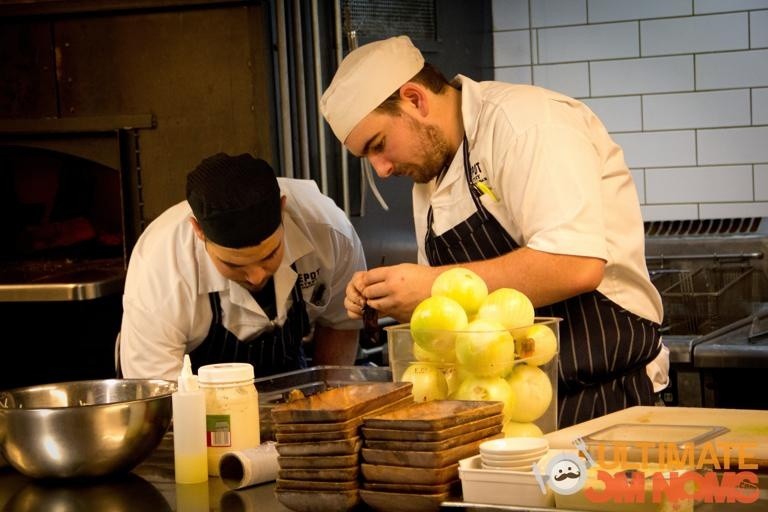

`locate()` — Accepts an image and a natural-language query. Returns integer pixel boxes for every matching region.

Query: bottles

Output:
[197,360,262,478]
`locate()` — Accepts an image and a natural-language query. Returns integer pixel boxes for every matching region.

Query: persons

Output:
[112,151,367,403]
[318,34,671,431]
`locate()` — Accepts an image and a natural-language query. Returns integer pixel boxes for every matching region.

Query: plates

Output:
[270,378,547,512]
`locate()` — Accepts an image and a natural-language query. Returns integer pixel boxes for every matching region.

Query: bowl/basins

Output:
[0,376,176,481]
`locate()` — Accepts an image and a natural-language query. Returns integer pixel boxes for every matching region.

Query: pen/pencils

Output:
[315,284,326,303]
[476,180,496,202]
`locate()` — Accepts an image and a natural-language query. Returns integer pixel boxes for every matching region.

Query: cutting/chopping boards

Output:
[543,401,768,469]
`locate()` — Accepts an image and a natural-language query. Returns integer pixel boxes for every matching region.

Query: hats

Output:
[319,34,425,144]
[186,150,283,249]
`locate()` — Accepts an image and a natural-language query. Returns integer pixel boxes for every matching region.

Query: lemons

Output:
[400,268,556,439]
